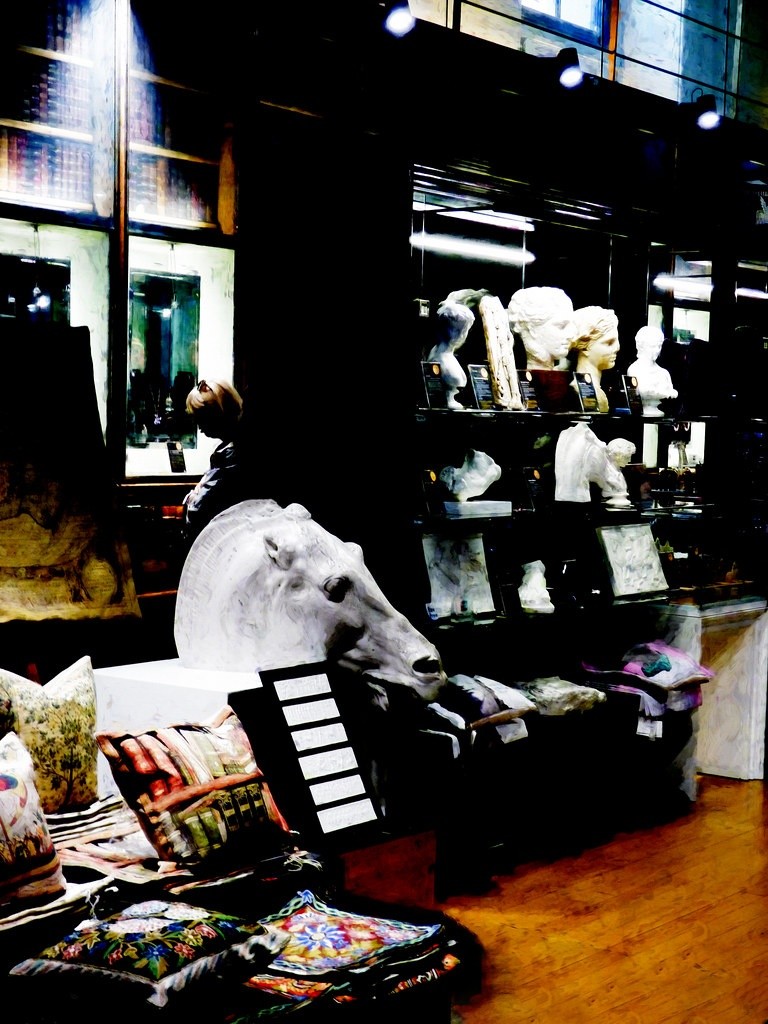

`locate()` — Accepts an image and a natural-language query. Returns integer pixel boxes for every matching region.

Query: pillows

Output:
[1,656,538,1024]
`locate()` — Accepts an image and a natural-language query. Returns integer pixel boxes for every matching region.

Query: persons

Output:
[181,380,244,517]
[602,438,636,508]
[427,304,475,409]
[505,287,678,417]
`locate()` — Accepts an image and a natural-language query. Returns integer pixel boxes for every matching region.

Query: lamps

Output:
[692,90,723,128]
[383,0,414,36]
[546,46,585,86]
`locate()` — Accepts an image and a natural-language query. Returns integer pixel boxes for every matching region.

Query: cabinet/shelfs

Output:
[410,410,689,738]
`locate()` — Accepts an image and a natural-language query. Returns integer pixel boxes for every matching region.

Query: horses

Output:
[555,423,627,502]
[172,498,448,701]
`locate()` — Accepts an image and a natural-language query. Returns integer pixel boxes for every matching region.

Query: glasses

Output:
[197,380,213,394]
